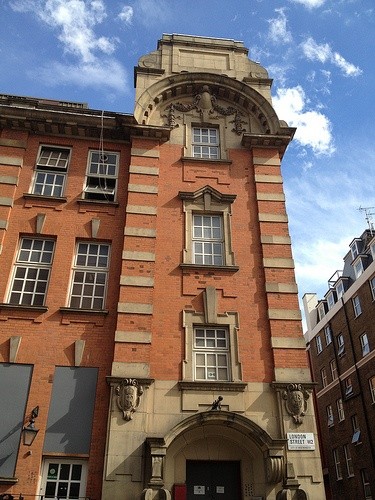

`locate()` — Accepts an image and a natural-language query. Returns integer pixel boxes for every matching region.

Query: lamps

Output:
[21,405,40,446]
